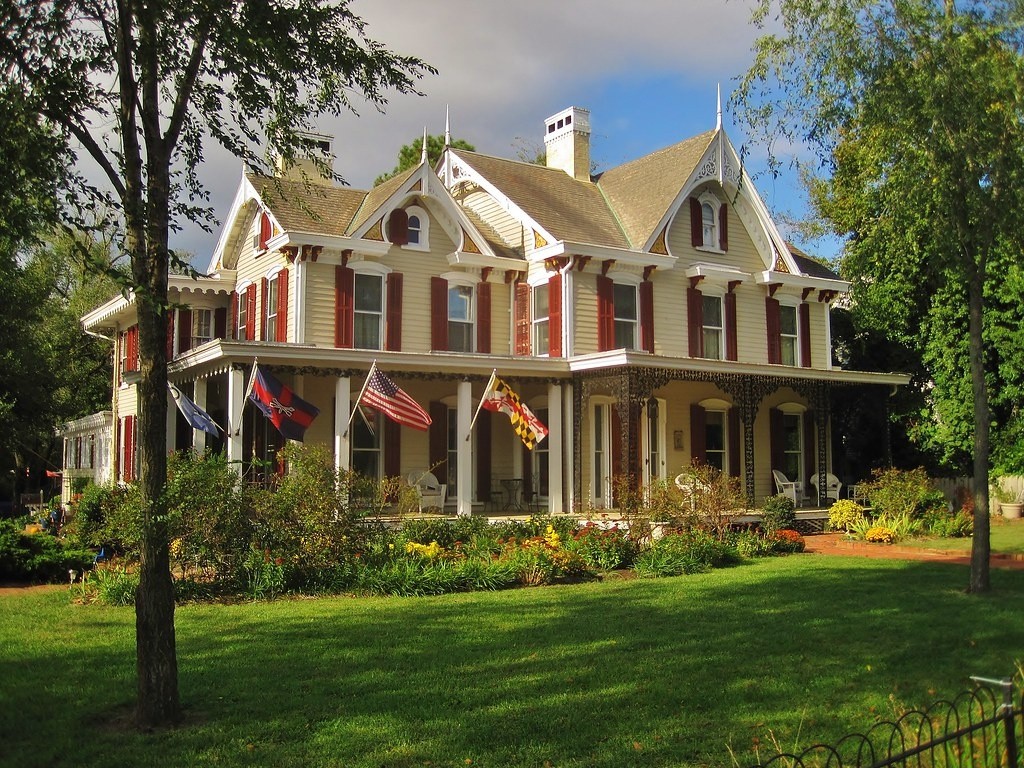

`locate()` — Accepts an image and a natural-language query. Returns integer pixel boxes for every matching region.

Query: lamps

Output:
[674,430,684,451]
[647,390,658,419]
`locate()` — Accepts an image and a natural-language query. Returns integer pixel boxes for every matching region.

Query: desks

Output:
[500,479,525,513]
[848,484,869,506]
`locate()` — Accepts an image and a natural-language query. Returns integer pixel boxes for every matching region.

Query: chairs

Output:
[772,469,803,509]
[476,471,503,513]
[675,473,712,512]
[408,470,447,513]
[810,473,842,507]
[519,471,542,513]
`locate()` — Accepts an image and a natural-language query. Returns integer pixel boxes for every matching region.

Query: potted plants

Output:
[996,488,1023,518]
[39,475,90,528]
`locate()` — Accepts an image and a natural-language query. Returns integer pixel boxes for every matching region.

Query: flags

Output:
[168,381,219,439]
[247,362,321,441]
[481,375,550,449]
[361,367,432,431]
